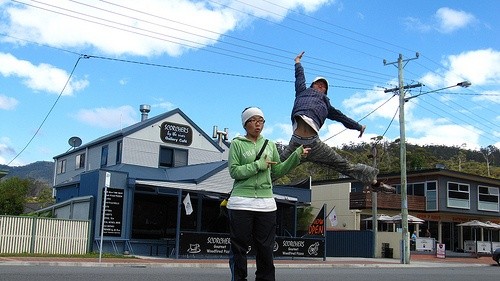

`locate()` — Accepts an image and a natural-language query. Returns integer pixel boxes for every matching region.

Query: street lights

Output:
[399,81,471,264]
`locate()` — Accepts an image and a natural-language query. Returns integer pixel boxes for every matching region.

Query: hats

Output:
[310,76,329,92]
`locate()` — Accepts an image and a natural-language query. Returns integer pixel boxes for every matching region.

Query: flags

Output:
[183,193,193,215]
[328,209,338,227]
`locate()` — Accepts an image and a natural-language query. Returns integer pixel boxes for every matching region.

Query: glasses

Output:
[248,119,264,124]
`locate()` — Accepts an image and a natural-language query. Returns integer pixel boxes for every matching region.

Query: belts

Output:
[293,134,317,140]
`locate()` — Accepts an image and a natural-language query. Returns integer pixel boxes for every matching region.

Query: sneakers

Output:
[371,181,396,193]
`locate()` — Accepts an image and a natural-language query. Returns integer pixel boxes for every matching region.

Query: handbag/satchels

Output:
[219,193,231,226]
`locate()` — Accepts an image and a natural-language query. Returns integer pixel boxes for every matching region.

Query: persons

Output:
[412,231,418,250]
[220,51,396,206]
[492,247,500,264]
[226,106,311,281]
[425,229,431,238]
